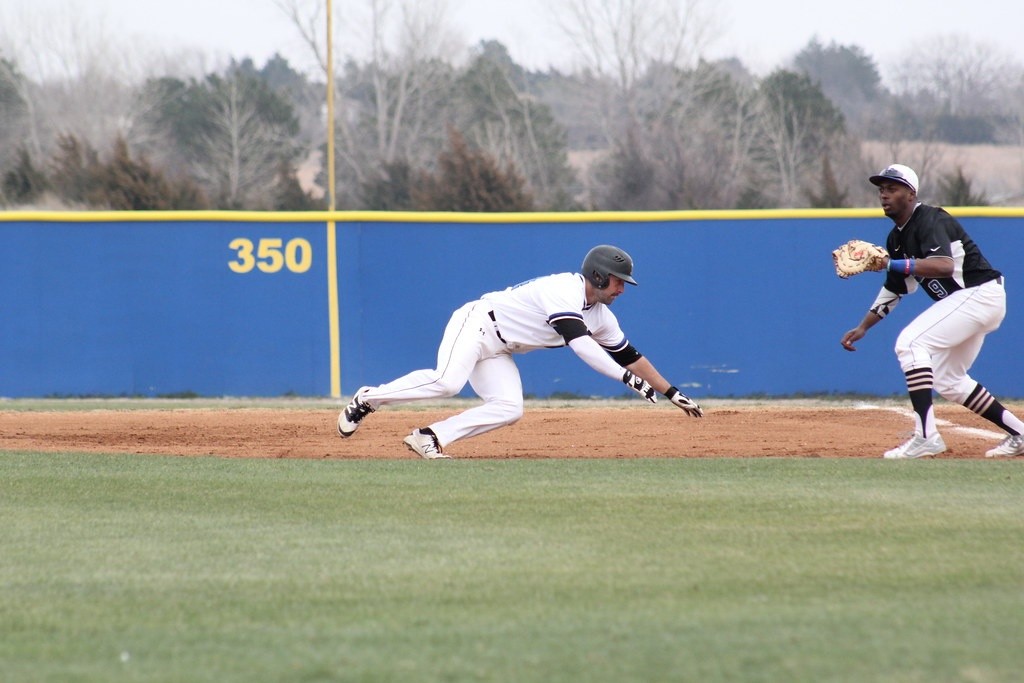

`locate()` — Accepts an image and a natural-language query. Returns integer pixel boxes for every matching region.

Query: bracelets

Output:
[887,258,915,275]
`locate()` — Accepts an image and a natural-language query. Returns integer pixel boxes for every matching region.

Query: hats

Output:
[869,164,918,198]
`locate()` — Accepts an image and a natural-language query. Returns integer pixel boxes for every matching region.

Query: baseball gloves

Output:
[831,239,889,280]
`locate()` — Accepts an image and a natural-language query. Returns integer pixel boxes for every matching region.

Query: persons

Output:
[839,163,1024,459]
[336,245,703,461]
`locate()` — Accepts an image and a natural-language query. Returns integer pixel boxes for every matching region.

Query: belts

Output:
[488,310,506,344]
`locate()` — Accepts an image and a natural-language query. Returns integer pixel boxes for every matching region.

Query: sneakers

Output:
[985,432,1024,459]
[882,430,947,461]
[401,429,453,460]
[337,385,380,438]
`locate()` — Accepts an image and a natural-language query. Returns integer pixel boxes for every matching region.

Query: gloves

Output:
[623,370,658,403]
[663,384,704,418]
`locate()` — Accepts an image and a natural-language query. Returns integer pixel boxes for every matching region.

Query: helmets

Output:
[580,244,638,290]
